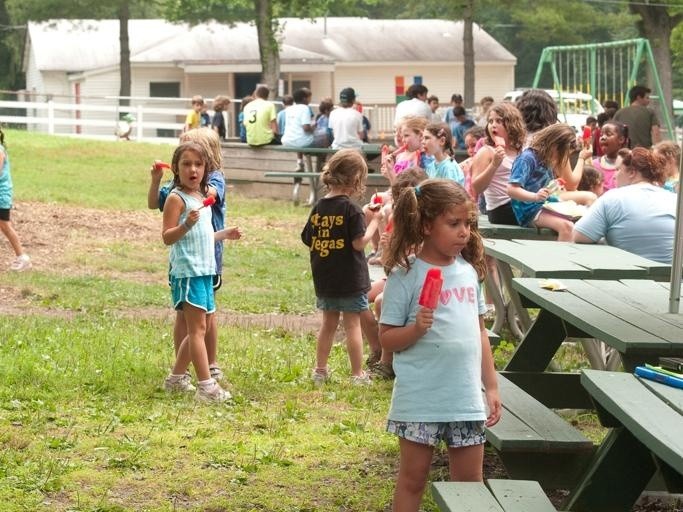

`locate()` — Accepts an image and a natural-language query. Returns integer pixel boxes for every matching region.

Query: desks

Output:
[566,369,682,511]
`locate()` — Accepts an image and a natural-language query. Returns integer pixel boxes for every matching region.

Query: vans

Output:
[501,87,604,128]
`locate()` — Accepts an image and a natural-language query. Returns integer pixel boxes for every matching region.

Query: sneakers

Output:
[295,162,305,172]
[163,363,234,404]
[312,353,394,387]
[11,255,32,272]
[484,308,516,320]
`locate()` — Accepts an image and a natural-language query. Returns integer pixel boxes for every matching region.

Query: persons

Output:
[378,180,502,511]
[148,128,226,381]
[0,130,33,274]
[382,84,682,265]
[162,142,244,403]
[300,149,385,384]
[188,85,371,155]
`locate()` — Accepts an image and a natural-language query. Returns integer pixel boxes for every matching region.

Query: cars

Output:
[653,98,682,145]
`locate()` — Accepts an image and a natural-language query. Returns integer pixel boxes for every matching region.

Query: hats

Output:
[340,88,356,100]
[452,94,463,102]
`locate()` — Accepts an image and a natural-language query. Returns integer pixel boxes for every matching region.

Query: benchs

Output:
[476,369,595,476]
[432,478,562,512]
